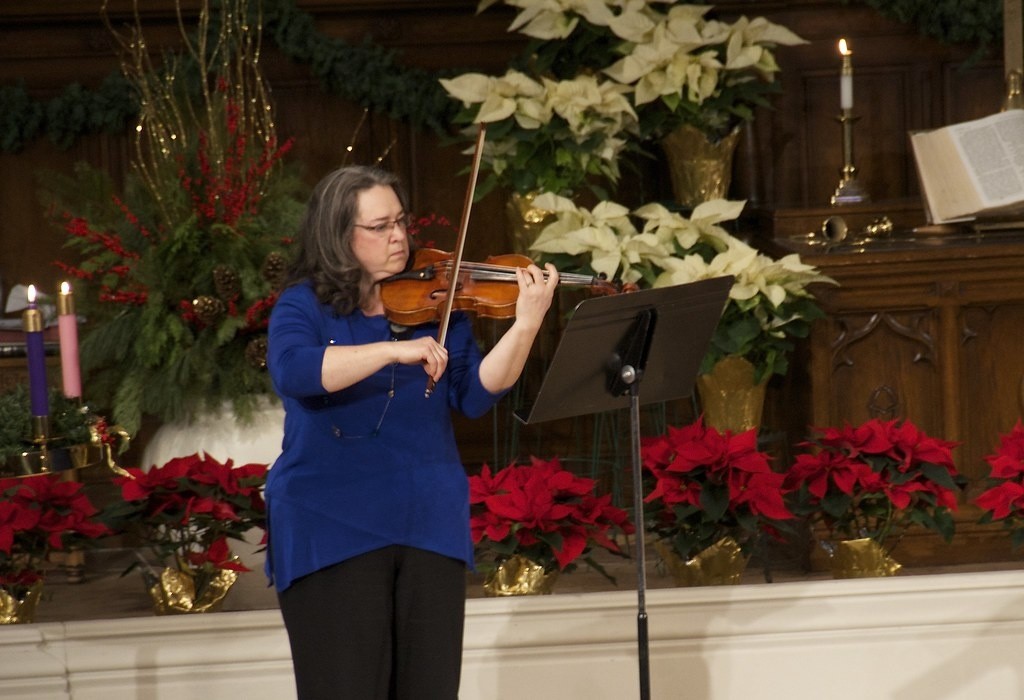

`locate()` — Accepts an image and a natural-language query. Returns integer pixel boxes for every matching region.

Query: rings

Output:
[527,280,533,286]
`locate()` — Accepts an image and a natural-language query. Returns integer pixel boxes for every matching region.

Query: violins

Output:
[379,245,641,327]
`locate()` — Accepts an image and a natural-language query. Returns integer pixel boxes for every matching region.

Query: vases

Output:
[139,393,287,575]
[145,554,239,613]
[696,356,769,436]
[825,539,902,580]
[485,556,560,597]
[659,119,742,208]
[660,532,758,587]
[0,577,45,624]
[506,186,581,257]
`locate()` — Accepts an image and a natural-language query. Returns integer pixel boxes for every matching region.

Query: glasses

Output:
[353,212,412,238]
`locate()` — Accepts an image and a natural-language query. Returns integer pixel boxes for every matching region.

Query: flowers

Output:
[49,76,460,456]
[467,453,636,585]
[0,470,119,600]
[969,416,1024,553]
[785,418,968,558]
[96,450,270,606]
[437,1,843,385]
[624,411,799,583]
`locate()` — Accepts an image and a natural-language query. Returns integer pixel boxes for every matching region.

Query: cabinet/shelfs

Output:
[760,200,1024,571]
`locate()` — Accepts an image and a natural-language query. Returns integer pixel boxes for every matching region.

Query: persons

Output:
[263,165,560,700]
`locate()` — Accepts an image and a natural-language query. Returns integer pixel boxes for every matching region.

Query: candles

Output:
[23,285,50,415]
[838,39,854,109]
[58,281,82,399]
